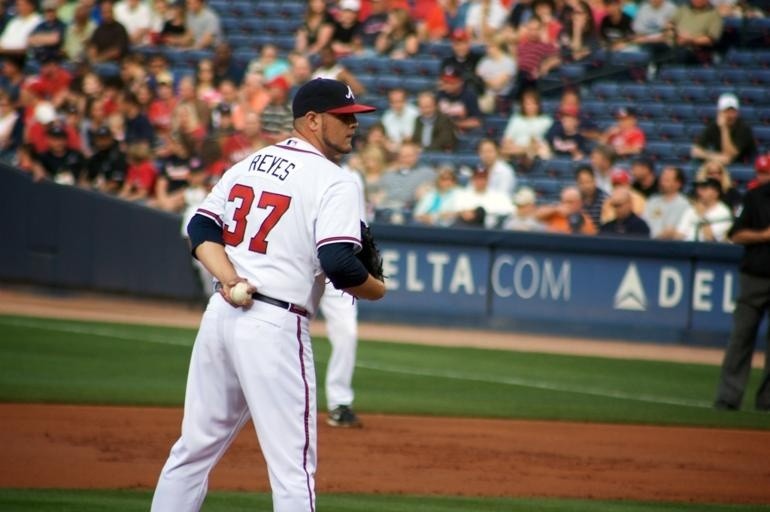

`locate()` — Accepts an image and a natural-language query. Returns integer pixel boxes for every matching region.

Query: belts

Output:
[215,281,307,317]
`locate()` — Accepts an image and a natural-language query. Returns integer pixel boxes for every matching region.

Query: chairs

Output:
[196,0,455,128]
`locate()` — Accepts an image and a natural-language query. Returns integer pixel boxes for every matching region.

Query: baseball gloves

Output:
[336,221,385,299]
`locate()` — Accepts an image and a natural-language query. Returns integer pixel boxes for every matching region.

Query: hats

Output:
[755,155,770,171]
[293,77,377,118]
[693,178,723,195]
[512,185,534,206]
[716,91,737,112]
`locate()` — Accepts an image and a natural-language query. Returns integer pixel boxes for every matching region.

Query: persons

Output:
[147,77,389,512]
[319,274,363,427]
[711,183,769,414]
[0,0,769,243]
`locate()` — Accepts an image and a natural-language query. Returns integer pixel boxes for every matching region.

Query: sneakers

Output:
[326,405,360,426]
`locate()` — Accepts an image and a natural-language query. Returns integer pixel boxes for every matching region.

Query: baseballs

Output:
[230,283,251,304]
